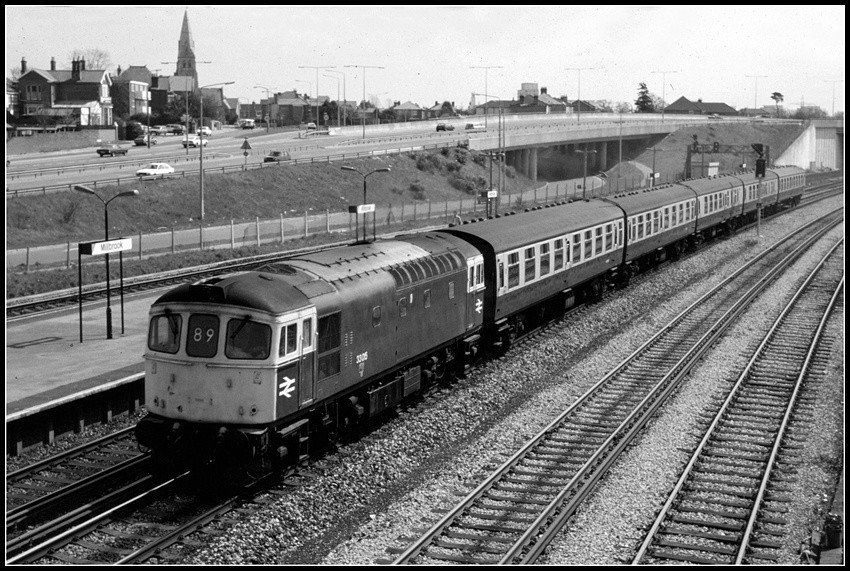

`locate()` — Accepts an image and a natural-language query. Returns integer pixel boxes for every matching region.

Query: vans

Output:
[465,122,486,128]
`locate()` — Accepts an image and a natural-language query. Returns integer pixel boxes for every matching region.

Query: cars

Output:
[97,143,128,157]
[242,119,257,130]
[134,134,158,146]
[141,124,212,137]
[708,113,723,119]
[136,162,175,176]
[182,136,209,148]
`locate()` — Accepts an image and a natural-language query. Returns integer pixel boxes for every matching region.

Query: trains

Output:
[127,164,808,504]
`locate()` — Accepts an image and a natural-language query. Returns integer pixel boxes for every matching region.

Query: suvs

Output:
[436,123,456,132]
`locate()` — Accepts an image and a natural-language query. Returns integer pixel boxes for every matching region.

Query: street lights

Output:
[824,78,843,115]
[646,145,664,187]
[564,67,595,121]
[575,147,597,198]
[253,86,270,116]
[339,165,392,242]
[264,150,291,162]
[470,64,504,115]
[294,64,347,128]
[73,184,140,339]
[199,79,236,220]
[161,60,212,157]
[649,69,677,124]
[478,151,505,219]
[344,64,385,139]
[745,74,768,119]
